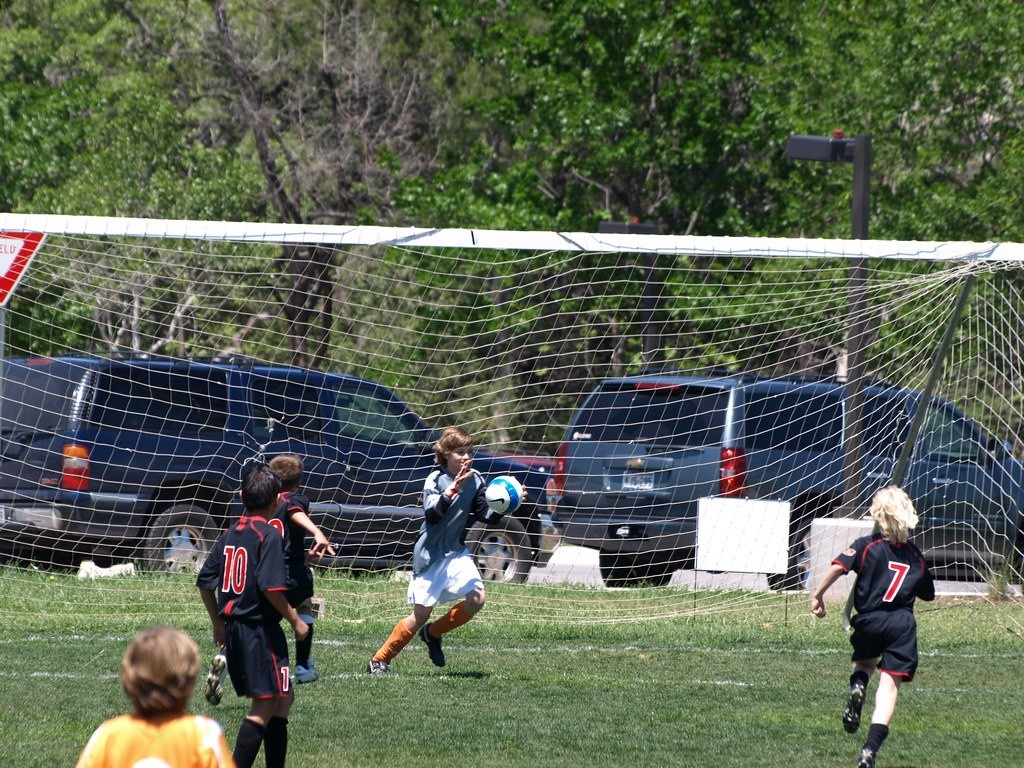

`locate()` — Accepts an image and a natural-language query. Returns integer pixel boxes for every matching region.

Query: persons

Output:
[368,427,528,677]
[196,454,336,768]
[810,485,935,768]
[74,628,237,768]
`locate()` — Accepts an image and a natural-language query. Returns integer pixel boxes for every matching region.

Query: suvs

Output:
[548,372,1024,589]
[1,350,546,586]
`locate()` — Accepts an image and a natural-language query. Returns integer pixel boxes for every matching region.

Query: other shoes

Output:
[857,748,877,768]
[842,682,865,734]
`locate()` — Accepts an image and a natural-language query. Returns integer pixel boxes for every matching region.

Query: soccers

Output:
[482,474,527,515]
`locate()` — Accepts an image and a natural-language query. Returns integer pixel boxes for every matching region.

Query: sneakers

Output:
[420,623,445,667]
[205,655,227,705]
[367,654,391,676]
[295,659,319,684]
